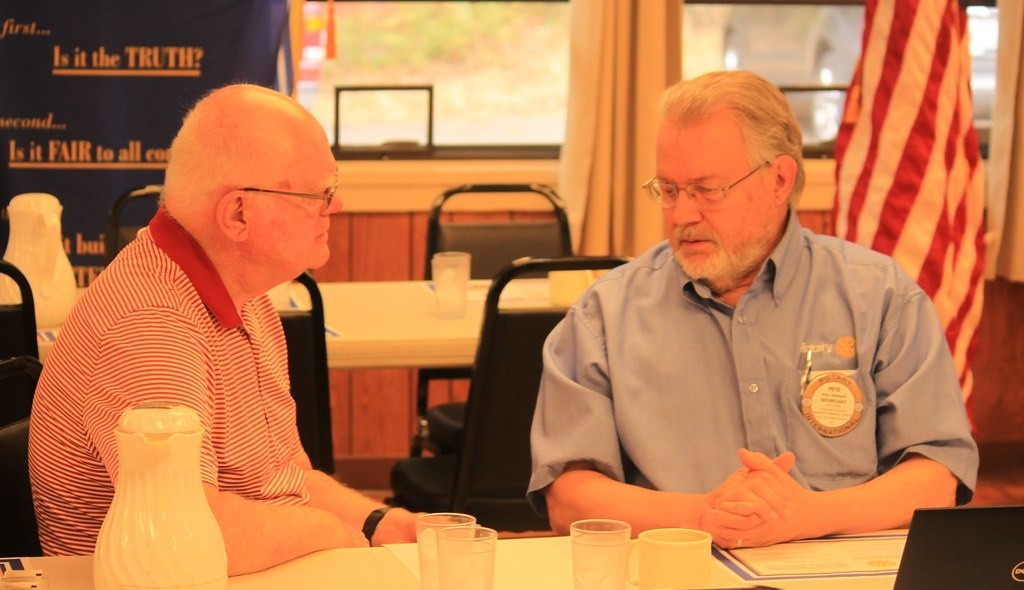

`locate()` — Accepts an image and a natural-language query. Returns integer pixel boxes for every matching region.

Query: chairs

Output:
[103,185,334,475]
[412,181,573,458]
[386,256,628,530]
[0,259,43,558]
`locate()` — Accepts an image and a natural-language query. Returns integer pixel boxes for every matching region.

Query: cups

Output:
[432,251,472,307]
[436,525,498,590]
[637,527,712,590]
[416,512,478,590]
[430,258,470,318]
[547,268,591,310]
[570,518,632,590]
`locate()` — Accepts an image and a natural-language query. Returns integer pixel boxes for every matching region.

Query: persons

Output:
[526,70,981,550]
[28,83,482,578]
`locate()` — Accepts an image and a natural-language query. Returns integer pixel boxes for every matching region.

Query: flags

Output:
[833,0,985,438]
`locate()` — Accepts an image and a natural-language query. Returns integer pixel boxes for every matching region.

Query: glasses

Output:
[642,155,781,210]
[239,187,337,215]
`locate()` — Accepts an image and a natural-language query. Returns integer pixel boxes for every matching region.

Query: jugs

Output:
[92,403,230,590]
[1,192,79,331]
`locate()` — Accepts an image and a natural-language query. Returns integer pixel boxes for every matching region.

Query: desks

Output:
[40,277,550,370]
[0,527,904,590]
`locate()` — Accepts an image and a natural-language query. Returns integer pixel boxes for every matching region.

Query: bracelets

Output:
[362,506,393,539]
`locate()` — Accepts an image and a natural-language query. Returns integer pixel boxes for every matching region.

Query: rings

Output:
[738,540,742,547]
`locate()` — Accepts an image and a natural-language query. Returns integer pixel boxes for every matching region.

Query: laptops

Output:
[893,505,1024,590]
